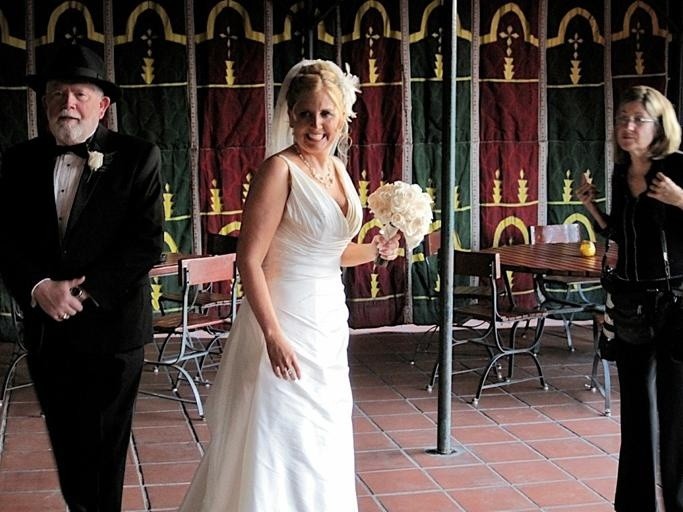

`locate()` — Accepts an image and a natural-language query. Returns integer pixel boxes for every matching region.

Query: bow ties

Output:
[56,143,89,159]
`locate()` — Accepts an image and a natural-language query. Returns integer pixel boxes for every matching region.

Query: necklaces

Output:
[293,142,334,190]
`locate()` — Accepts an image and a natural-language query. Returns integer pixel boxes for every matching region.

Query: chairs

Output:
[423,224,615,418]
[143,231,240,419]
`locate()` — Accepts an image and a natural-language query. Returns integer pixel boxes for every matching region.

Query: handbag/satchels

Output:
[597,266,683,364]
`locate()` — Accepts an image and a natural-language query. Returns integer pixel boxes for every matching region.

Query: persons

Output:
[575,86,682,512]
[1,81,166,512]
[177,60,401,512]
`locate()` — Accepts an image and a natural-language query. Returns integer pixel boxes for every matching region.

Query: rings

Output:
[583,192,591,197]
[286,368,294,375]
[58,313,67,319]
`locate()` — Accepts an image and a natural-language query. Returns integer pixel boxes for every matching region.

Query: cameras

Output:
[646,289,683,323]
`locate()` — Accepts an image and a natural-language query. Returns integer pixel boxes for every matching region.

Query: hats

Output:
[26,42,122,104]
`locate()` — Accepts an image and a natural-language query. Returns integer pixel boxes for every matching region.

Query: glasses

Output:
[614,115,654,125]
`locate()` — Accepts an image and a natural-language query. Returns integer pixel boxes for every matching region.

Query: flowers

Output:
[367,179,435,267]
[85,148,109,181]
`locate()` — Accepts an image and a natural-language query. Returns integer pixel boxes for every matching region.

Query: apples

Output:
[579,240,595,256]
